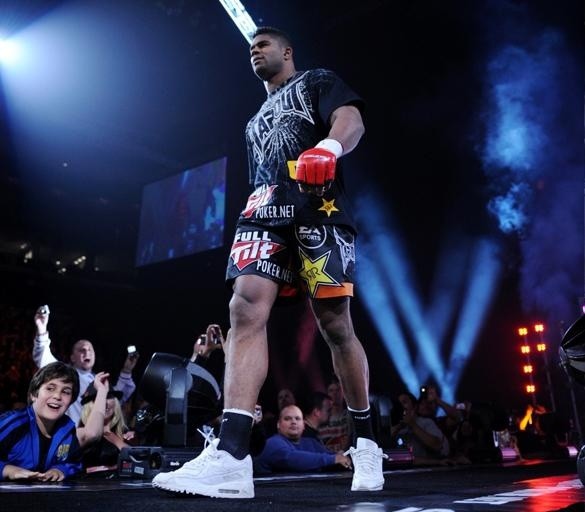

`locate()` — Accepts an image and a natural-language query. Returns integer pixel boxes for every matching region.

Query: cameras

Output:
[40,304,50,316]
[455,403,465,410]
[127,345,137,358]
[420,387,427,400]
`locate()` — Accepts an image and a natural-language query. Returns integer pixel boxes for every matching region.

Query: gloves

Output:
[295,139,343,197]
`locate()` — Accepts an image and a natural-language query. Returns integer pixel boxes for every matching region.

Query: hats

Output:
[80,381,123,405]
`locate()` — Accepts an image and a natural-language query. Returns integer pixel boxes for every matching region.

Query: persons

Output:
[152,28,385,499]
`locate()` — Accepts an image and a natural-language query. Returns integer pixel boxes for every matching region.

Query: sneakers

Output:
[349,437,385,491]
[151,438,255,498]
